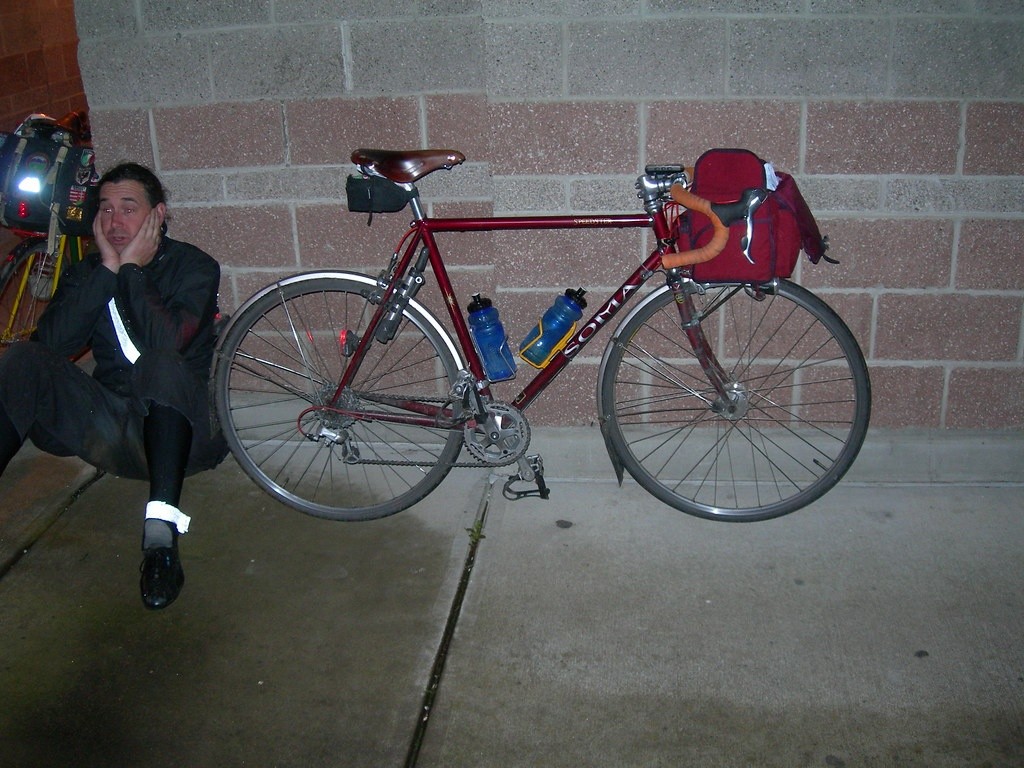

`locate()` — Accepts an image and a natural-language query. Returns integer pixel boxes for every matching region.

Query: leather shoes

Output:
[136,526,185,609]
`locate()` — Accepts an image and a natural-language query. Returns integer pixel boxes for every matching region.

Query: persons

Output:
[1,163,231,608]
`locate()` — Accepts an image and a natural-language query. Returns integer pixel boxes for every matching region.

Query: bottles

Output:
[467,293,516,380]
[520,286,585,364]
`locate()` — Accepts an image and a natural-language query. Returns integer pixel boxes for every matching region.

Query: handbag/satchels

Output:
[0,128,100,237]
[687,149,830,279]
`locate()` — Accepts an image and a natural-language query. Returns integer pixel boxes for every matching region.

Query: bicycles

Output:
[209,150,873,523]
[0,111,98,351]
